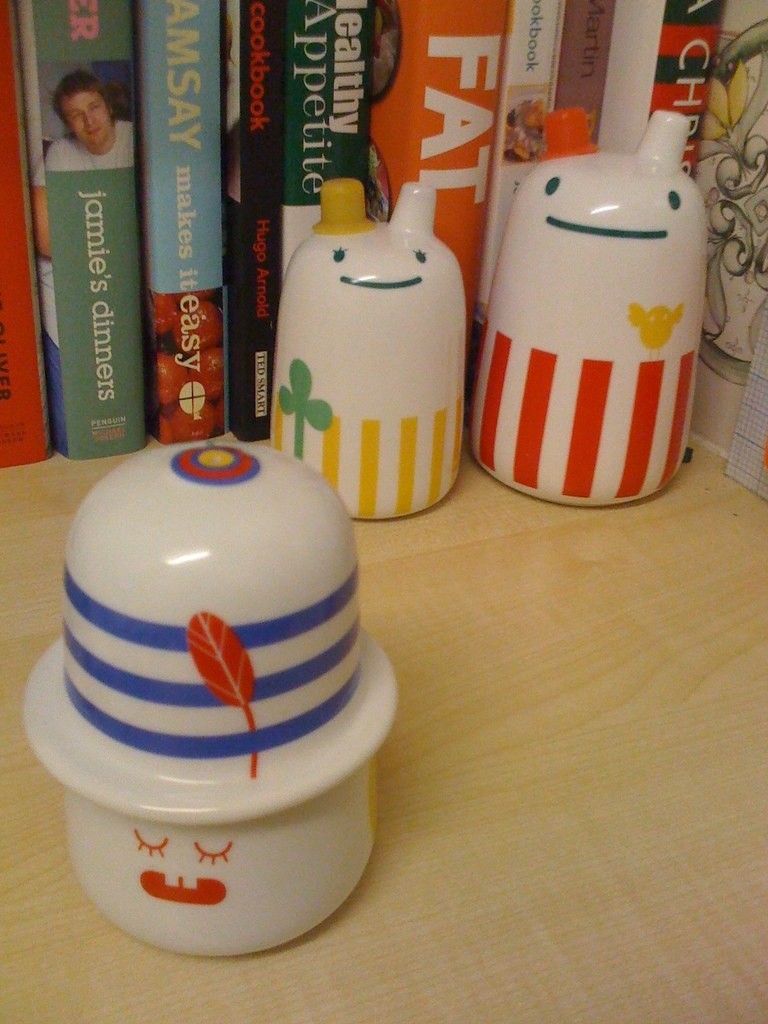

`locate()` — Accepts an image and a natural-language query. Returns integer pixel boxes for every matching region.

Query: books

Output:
[1,0,723,469]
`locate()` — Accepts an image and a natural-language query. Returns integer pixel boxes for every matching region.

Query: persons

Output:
[32,69,133,257]
